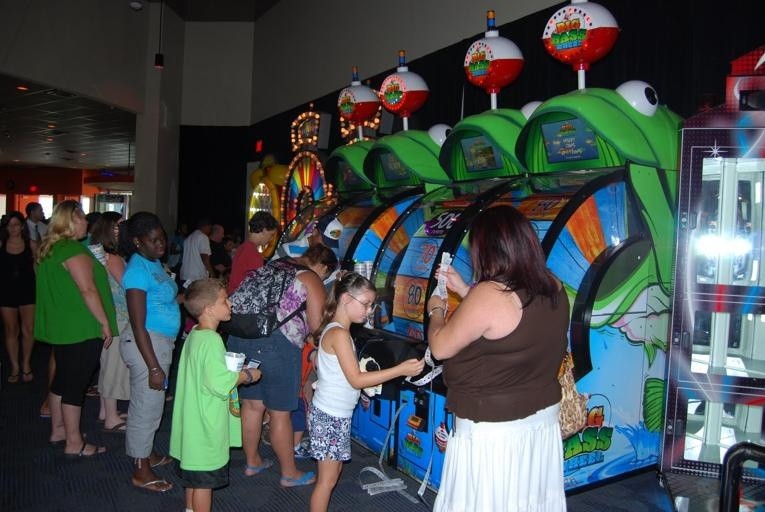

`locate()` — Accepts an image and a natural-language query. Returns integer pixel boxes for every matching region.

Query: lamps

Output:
[153,0,165,71]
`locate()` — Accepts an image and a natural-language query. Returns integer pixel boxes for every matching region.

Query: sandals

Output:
[165,392,173,401]
[244,458,275,477]
[279,471,317,488]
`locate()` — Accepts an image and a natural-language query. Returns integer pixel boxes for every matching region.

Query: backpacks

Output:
[166,243,183,273]
[220,254,312,339]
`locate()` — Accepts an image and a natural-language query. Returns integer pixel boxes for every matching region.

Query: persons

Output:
[228,242,339,491]
[115,209,178,494]
[426,201,570,512]
[305,270,425,509]
[166,275,262,510]
[1,200,320,461]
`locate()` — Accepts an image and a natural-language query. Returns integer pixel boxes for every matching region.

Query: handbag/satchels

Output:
[556,352,589,439]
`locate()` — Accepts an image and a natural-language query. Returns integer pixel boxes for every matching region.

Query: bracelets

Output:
[428,306,445,320]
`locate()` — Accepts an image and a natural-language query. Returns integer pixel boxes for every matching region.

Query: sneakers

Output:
[85,385,101,400]
[261,421,272,446]
[294,445,315,458]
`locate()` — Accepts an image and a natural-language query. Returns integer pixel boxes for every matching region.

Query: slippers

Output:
[63,443,107,459]
[102,421,127,433]
[133,479,175,495]
[150,455,177,469]
[40,412,52,423]
[96,411,128,423]
[48,432,87,446]
[7,373,20,385]
[22,369,35,383]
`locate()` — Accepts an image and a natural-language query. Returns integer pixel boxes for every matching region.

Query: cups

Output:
[88,242,106,266]
[224,351,246,372]
[354,262,367,278]
[362,261,373,281]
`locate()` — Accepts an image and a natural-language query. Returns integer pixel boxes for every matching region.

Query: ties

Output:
[34,225,43,246]
